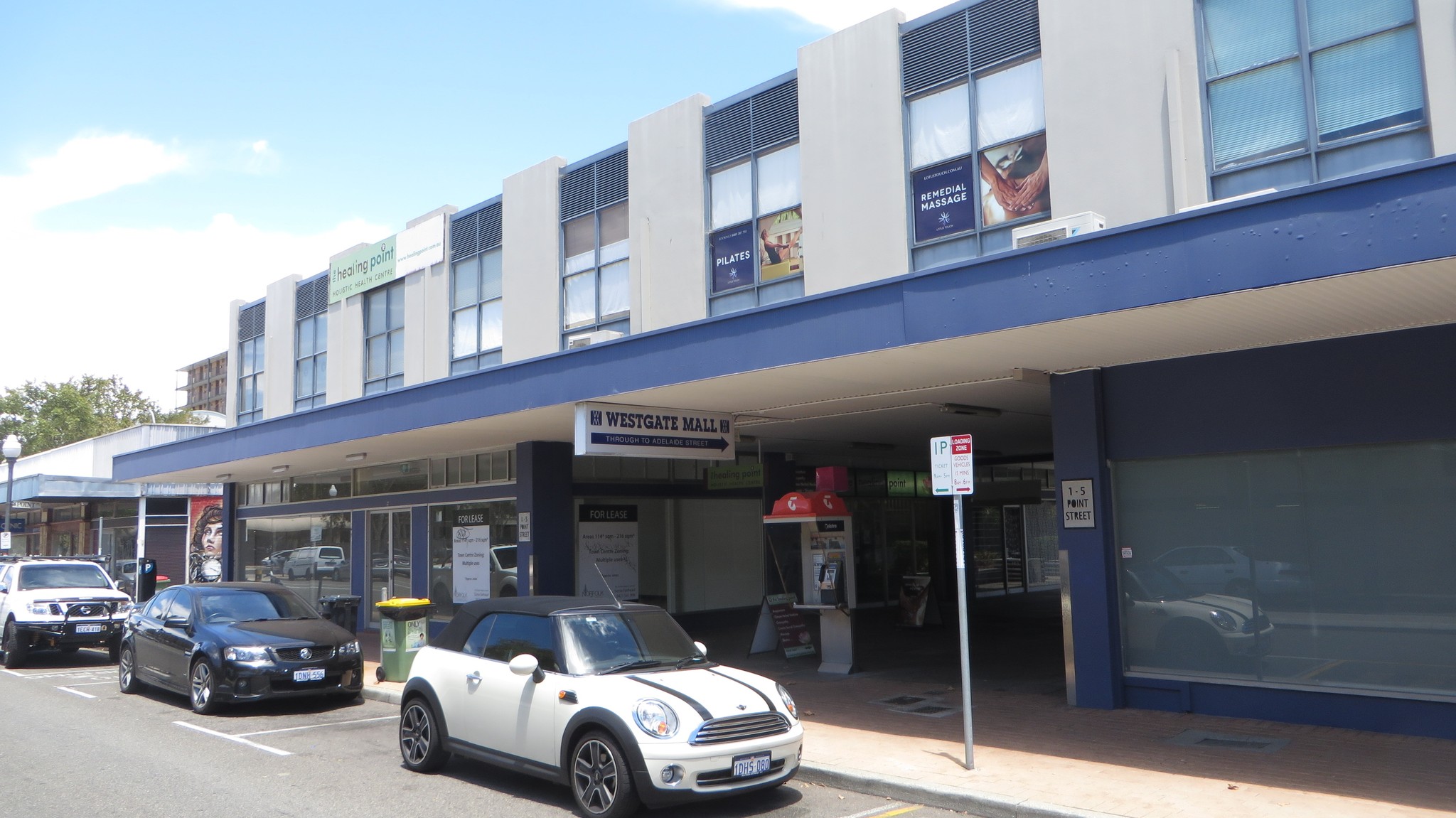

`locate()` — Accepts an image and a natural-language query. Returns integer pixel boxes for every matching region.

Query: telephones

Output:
[818,561,843,603]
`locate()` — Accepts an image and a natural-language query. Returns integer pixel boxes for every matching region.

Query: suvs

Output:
[0,551,138,669]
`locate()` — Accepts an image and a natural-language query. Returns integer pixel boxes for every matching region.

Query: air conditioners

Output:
[1012,212,1106,250]
[568,330,624,350]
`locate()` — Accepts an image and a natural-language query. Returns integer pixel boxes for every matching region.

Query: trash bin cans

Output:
[374,598,436,682]
[317,596,362,634]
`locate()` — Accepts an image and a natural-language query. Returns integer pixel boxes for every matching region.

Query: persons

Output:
[789,226,803,258]
[761,229,790,265]
[980,137,1049,226]
[898,578,931,624]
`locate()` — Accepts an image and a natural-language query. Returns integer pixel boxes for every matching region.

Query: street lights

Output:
[1,434,22,554]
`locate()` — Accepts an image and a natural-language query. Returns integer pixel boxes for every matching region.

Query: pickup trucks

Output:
[431,541,533,614]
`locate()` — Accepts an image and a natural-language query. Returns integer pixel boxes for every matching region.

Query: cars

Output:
[399,596,806,818]
[261,546,411,583]
[1119,553,1276,673]
[118,581,366,717]
[1150,545,1304,602]
